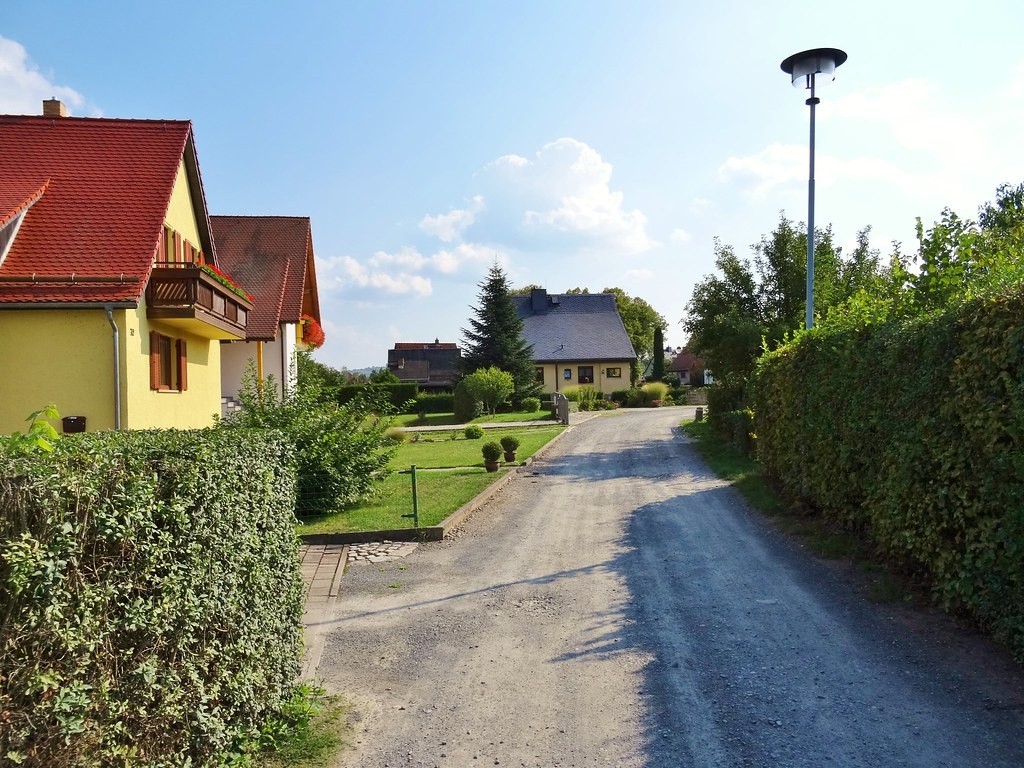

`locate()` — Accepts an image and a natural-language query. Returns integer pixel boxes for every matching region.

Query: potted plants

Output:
[500,435,521,462]
[481,441,503,473]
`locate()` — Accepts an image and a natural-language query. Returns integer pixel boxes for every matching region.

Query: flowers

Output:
[196,259,255,305]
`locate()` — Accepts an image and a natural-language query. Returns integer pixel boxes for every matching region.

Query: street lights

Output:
[780,47,847,329]
